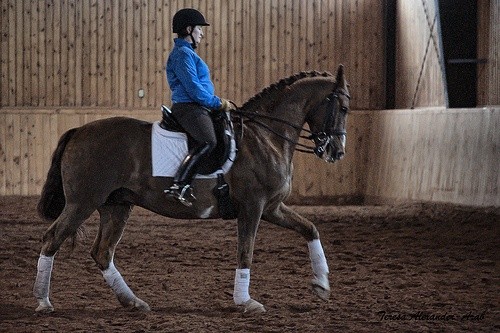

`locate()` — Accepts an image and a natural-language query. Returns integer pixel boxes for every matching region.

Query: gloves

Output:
[221,99,237,111]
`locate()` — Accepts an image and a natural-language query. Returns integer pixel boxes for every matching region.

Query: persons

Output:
[167,8,232,204]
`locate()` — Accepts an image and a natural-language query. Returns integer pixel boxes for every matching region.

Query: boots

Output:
[166,142,213,201]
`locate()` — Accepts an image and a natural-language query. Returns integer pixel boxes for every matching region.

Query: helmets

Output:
[172,8,210,34]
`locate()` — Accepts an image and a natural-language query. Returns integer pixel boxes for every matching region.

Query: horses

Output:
[33,64,352,318]
